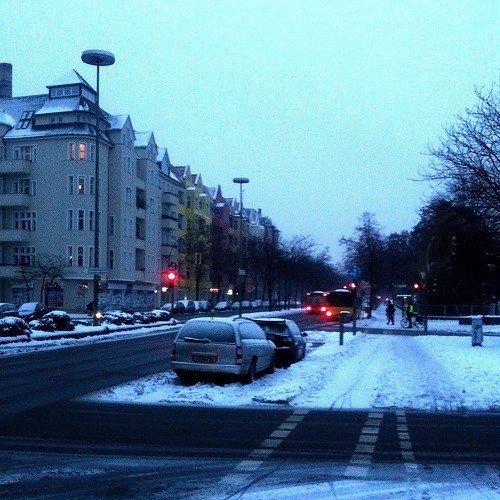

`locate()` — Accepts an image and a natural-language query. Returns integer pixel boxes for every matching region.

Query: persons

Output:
[386,301,396,325]
[406,300,413,328]
[86,301,94,316]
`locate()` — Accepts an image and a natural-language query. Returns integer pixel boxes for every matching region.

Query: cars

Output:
[0,302,49,322]
[160,300,212,315]
[170,315,308,385]
[215,300,302,311]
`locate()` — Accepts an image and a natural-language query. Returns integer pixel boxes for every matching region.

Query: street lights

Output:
[349,279,356,335]
[167,272,175,314]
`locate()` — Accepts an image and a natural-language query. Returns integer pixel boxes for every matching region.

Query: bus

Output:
[306,289,353,319]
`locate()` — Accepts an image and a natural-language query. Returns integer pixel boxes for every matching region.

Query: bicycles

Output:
[400,313,424,328]
